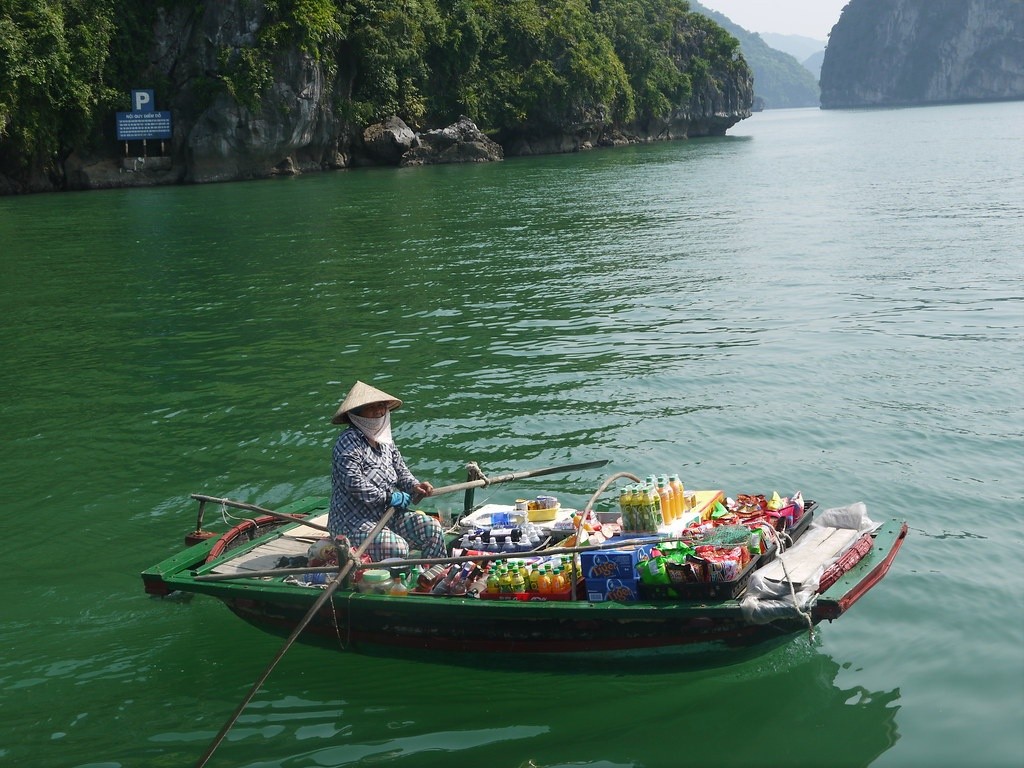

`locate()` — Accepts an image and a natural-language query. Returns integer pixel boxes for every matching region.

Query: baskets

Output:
[480,577,584,602]
[407,581,468,598]
[750,538,787,569]
[446,532,555,553]
[775,500,818,548]
[638,553,762,600]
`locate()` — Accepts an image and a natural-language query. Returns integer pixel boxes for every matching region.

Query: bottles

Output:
[356,472,695,602]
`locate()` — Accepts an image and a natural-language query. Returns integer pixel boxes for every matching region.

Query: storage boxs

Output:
[407,491,818,601]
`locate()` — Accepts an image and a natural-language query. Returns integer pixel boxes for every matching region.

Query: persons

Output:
[326,381,449,568]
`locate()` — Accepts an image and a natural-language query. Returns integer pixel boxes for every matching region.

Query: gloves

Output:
[385,492,412,510]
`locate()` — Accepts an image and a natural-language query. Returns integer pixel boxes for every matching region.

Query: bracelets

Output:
[385,492,392,505]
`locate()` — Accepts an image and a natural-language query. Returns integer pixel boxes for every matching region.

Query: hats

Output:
[331,381,403,425]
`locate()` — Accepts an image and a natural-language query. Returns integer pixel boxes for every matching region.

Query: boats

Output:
[140,494,909,662]
